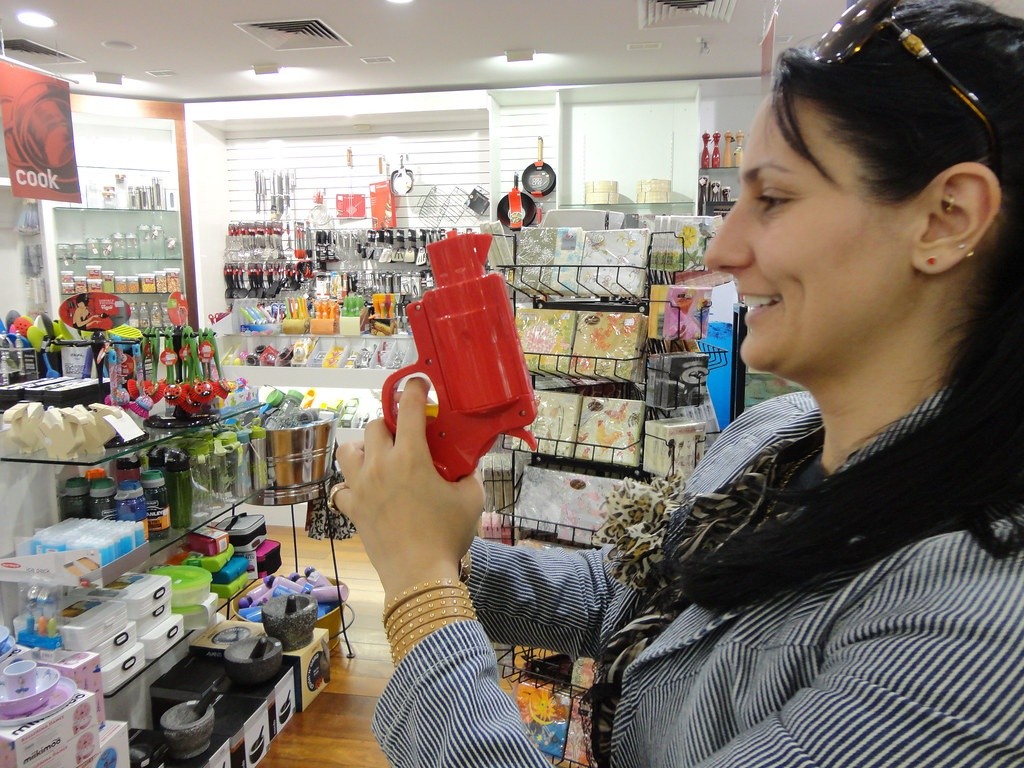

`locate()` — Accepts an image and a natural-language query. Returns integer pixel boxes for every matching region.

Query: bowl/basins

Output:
[232,575,350,657]
[266,409,341,489]
[0,666,61,716]
[0,624,10,652]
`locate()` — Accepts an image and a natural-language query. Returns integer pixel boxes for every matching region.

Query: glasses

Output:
[813,0,1003,182]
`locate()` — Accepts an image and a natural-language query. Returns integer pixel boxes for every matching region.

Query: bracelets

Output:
[382,578,477,668]
[459,552,471,584]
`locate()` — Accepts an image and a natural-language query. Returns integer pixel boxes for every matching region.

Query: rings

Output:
[327,485,348,515]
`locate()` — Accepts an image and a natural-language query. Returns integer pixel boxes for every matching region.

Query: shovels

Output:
[365,229,448,265]
[247,263,265,299]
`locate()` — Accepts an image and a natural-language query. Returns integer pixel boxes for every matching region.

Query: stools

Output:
[227,480,354,658]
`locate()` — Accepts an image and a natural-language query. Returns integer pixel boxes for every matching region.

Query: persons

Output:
[329,0,1024,768]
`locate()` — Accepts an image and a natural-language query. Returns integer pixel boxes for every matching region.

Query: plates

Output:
[0,635,16,662]
[0,676,77,727]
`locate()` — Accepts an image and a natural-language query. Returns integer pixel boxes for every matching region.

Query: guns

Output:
[380,232,543,483]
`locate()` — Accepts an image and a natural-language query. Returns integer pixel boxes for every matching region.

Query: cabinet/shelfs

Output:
[493,226,726,768]
[1,403,264,700]
[51,207,184,330]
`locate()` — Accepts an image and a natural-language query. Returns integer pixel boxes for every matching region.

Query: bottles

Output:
[57,172,182,329]
[60,389,336,543]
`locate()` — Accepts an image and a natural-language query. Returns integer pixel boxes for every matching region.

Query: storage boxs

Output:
[0,514,331,768]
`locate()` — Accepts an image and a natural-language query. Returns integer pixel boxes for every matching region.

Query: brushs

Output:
[104,325,237,419]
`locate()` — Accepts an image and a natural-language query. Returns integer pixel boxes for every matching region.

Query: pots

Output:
[390,155,414,195]
[497,135,556,231]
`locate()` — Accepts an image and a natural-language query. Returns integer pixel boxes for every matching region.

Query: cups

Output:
[3,659,37,699]
[238,566,349,624]
